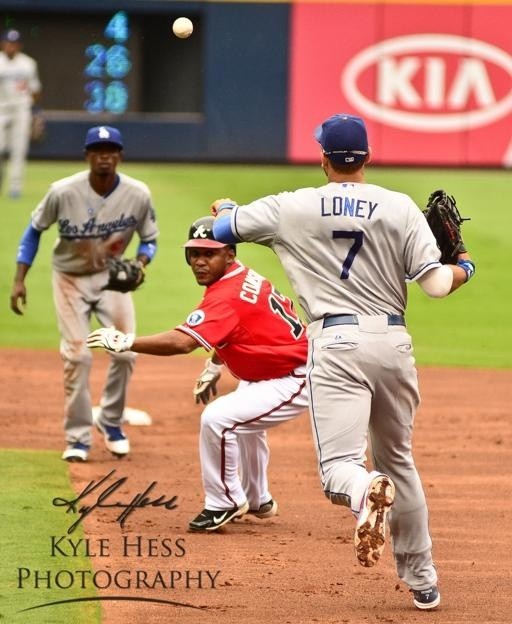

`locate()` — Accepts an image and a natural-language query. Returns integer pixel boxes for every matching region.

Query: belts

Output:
[321,315,406,327]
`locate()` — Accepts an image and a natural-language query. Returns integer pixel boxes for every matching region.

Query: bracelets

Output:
[456,259,475,283]
[216,203,236,210]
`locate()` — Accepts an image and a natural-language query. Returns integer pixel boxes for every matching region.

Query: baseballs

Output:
[172,16,193,37]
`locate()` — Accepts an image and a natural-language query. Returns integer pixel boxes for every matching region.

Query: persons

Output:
[0,31,42,199]
[209,114,475,609]
[9,126,157,460]
[85,216,310,535]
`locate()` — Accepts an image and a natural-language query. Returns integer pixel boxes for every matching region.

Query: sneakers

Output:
[188,501,249,530]
[62,442,88,461]
[250,500,277,517]
[353,476,396,568]
[409,585,440,611]
[97,419,130,454]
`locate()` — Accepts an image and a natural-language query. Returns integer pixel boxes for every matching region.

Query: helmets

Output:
[184,215,237,264]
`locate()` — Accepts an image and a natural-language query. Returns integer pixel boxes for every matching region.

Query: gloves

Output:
[88,328,133,352]
[194,360,224,403]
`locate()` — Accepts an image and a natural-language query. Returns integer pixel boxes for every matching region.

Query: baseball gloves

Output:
[99,256,146,292]
[422,190,467,265]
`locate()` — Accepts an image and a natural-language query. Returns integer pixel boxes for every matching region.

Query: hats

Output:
[84,126,124,148]
[315,114,370,156]
